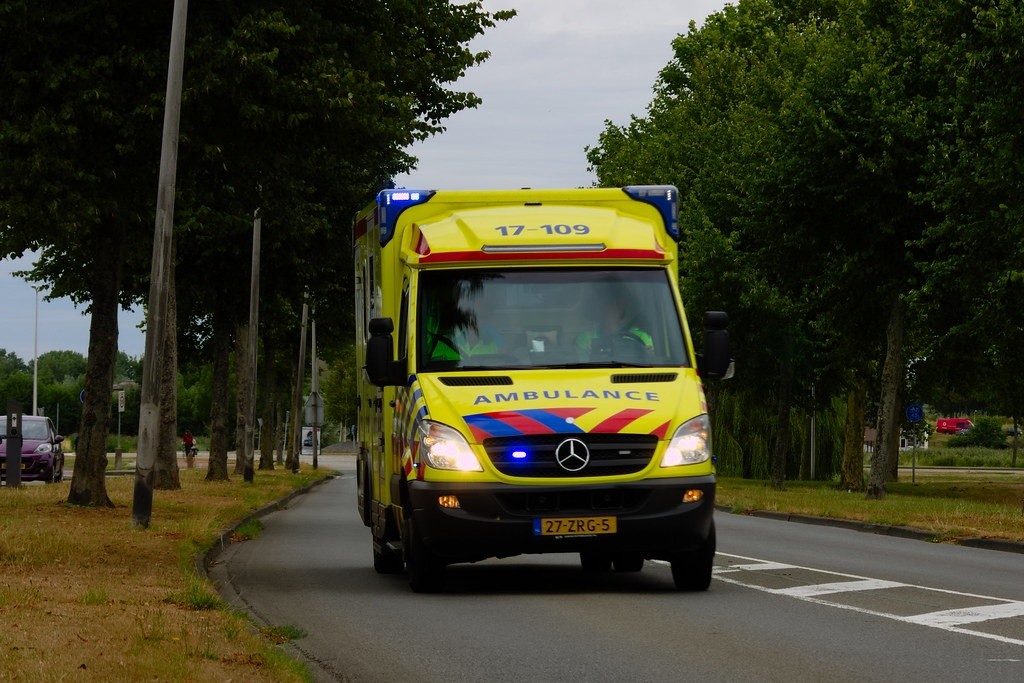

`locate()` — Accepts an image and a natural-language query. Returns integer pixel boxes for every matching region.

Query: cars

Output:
[1007,426,1024,437]
[0,415,65,486]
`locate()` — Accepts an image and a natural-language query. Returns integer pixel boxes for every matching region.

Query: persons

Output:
[574,296,655,354]
[430,298,500,358]
[181,429,198,457]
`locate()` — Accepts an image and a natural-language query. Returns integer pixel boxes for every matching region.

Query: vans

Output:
[936,418,981,437]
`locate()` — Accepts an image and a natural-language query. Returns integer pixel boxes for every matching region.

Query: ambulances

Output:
[355,185,736,594]
